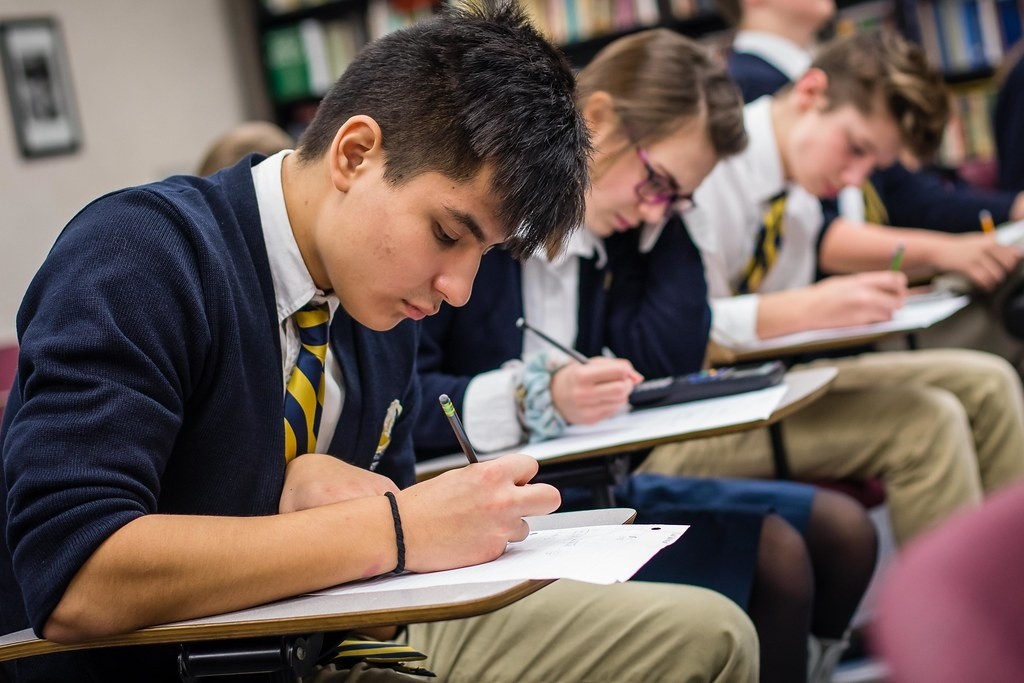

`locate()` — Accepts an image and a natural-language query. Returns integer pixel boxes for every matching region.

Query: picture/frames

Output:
[0,18,82,158]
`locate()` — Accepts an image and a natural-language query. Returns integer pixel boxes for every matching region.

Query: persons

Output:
[0,0,1024,683]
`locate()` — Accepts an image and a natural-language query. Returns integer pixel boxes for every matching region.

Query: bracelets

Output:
[385,492,405,574]
[525,352,567,444]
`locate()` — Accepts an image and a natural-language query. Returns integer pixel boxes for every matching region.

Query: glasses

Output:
[623,119,695,218]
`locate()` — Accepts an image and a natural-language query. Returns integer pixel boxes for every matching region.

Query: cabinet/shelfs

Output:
[248,0,1024,190]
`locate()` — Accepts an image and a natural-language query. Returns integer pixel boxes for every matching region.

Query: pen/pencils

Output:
[889,244,906,270]
[979,209,994,233]
[438,393,479,464]
[515,317,589,364]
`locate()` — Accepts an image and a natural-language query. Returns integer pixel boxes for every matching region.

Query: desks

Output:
[0,506,639,683]
[411,366,839,481]
[704,295,970,367]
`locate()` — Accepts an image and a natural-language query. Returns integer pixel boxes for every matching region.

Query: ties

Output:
[733,191,789,296]
[283,300,429,660]
